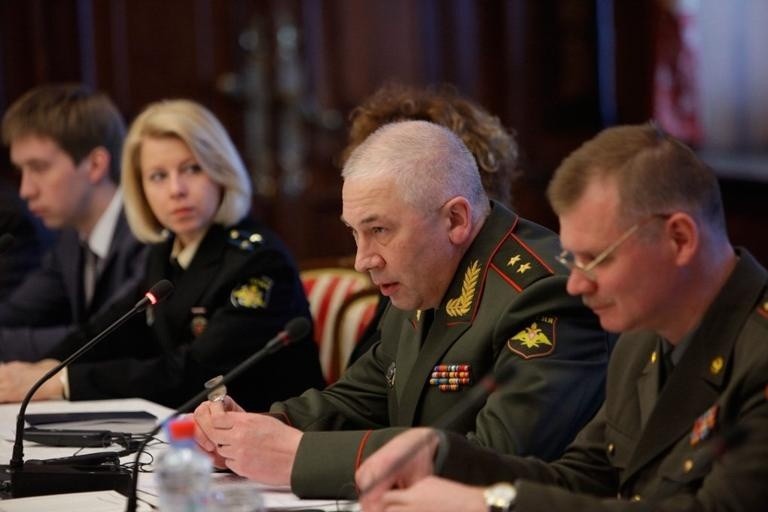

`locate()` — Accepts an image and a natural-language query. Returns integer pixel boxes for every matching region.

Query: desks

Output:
[0,386,370,512]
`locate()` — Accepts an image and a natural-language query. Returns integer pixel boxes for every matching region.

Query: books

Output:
[19,411,158,435]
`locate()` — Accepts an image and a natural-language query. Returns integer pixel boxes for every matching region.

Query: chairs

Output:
[295,264,385,385]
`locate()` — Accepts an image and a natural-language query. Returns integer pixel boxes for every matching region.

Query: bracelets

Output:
[62,364,69,397]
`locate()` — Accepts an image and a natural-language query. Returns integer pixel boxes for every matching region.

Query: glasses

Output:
[552,212,672,286]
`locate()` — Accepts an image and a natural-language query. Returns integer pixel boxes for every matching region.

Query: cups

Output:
[194,476,263,511]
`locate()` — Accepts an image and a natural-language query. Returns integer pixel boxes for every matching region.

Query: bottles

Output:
[150,420,216,511]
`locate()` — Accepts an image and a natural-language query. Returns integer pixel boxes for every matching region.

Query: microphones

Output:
[0,280,175,498]
[128,318,310,512]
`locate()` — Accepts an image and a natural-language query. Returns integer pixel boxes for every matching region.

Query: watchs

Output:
[485,480,516,512]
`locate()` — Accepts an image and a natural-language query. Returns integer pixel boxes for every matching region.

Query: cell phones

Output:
[23,426,114,447]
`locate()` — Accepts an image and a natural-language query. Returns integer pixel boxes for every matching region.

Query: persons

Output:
[356,124,767,512]
[0,84,146,354]
[1,99,327,406]
[192,119,620,499]
[347,81,526,368]
